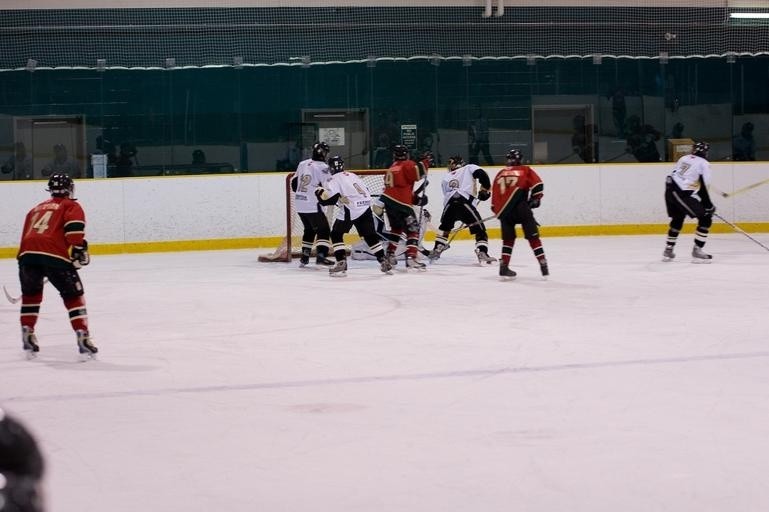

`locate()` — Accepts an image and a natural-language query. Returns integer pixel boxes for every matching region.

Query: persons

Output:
[16,171,98,360]
[0,142,32,180]
[42,144,81,178]
[0,409,46,511]
[662,141,716,265]
[362,81,756,161]
[291,141,547,274]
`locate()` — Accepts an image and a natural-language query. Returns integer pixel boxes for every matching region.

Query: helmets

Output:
[312,141,330,161]
[328,155,345,176]
[505,148,524,166]
[392,144,410,161]
[447,155,466,172]
[692,140,710,159]
[45,172,76,198]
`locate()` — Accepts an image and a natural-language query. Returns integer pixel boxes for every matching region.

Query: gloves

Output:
[413,194,428,207]
[70,239,90,267]
[421,150,435,165]
[528,198,541,207]
[477,190,491,201]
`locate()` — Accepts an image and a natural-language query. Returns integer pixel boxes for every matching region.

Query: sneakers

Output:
[380,250,397,272]
[299,253,311,264]
[427,251,442,261]
[328,259,348,273]
[663,246,676,258]
[406,256,427,269]
[316,254,335,265]
[474,248,496,261]
[692,244,712,259]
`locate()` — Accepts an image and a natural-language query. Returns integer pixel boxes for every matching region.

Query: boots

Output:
[75,329,99,354]
[498,259,517,277]
[540,259,549,276]
[21,325,40,353]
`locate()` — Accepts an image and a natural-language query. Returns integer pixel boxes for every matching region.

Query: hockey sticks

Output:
[416,173,497,260]
[3,258,80,304]
[706,180,768,198]
